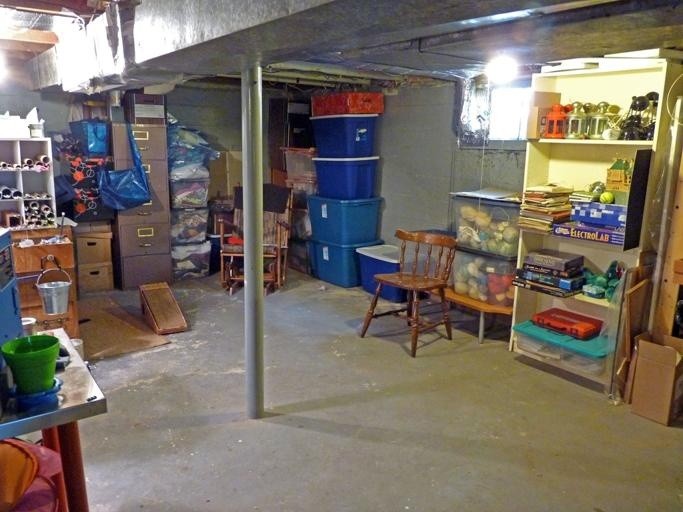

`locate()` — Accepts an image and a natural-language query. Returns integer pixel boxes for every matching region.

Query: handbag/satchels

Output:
[96,166,151,210]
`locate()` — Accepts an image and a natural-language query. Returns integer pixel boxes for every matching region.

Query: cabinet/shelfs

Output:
[0,138,57,231]
[508,60,683,399]
[10,225,81,340]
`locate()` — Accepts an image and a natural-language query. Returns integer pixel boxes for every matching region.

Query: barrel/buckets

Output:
[2,334,60,394]
[35,268,71,316]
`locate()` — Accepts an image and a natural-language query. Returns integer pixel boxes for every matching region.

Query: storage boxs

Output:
[631,332,683,426]
[271,92,434,303]
[451,197,520,257]
[75,92,212,292]
[452,246,517,307]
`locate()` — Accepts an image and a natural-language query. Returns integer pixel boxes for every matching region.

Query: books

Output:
[511,180,601,237]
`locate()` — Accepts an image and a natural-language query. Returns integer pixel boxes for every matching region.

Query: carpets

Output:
[77,296,170,361]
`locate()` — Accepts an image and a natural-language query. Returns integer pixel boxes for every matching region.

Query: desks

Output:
[0,328,108,512]
[431,287,513,344]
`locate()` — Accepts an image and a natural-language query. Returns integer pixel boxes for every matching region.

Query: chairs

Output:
[218,183,293,296]
[361,228,458,358]
